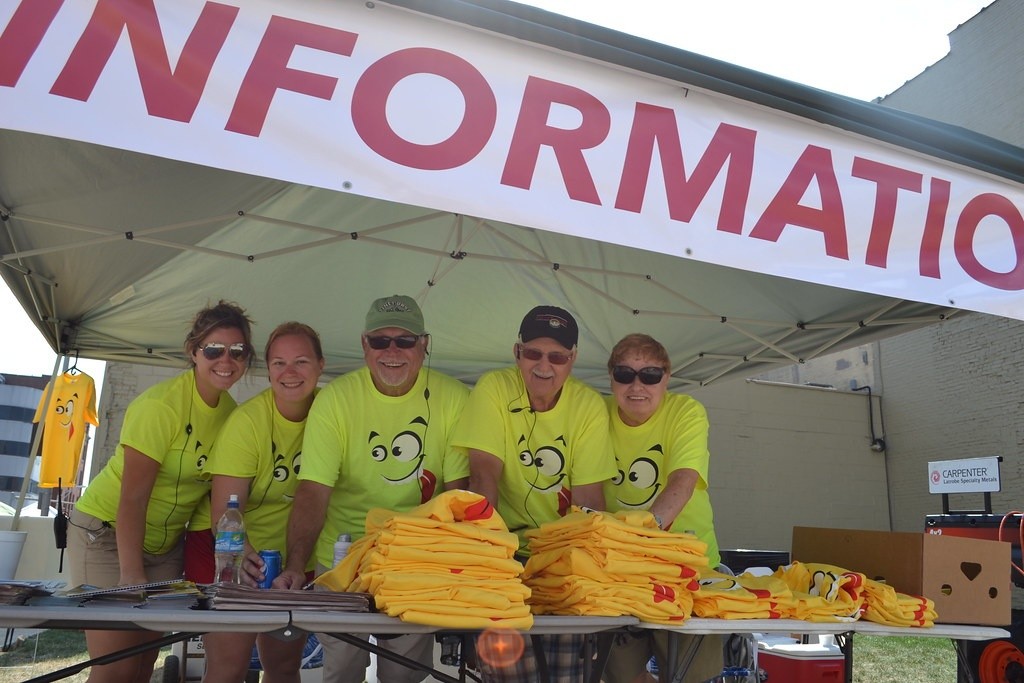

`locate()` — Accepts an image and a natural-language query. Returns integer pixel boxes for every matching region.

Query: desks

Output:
[0,594,1012,683]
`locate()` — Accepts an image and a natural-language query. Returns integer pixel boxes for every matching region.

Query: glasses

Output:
[197,341,251,362]
[519,338,575,365]
[366,334,423,350]
[610,363,668,385]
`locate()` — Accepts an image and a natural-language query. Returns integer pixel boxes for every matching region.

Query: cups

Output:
[0,531,28,580]
[818,634,834,646]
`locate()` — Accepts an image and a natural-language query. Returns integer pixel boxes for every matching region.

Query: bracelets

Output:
[653,514,663,529]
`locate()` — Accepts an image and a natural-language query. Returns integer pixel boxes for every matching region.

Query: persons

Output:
[68,303,256,683]
[200,321,326,683]
[450,305,618,683]
[273,295,472,683]
[600,334,724,683]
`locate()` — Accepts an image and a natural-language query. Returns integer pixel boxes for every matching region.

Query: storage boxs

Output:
[790,525,1012,628]
[755,636,845,682]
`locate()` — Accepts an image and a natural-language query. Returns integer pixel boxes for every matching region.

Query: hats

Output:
[518,305,578,351]
[365,294,426,336]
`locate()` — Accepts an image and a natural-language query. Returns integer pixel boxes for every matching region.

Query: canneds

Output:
[255,549,283,590]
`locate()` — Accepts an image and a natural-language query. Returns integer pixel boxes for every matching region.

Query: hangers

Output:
[63,348,82,375]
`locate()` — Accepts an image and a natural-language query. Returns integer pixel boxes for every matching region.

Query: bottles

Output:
[215,495,246,584]
[333,535,353,569]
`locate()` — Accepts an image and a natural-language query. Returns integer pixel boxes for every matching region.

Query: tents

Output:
[0,0,1024,531]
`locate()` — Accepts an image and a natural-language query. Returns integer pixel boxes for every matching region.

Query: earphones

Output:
[192,349,196,356]
